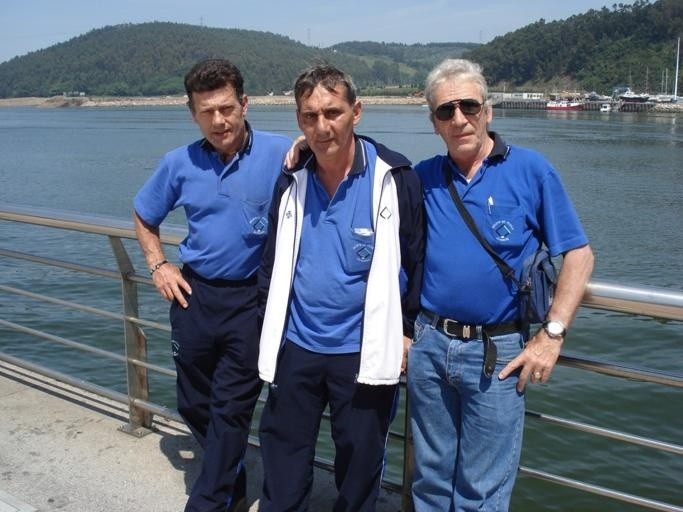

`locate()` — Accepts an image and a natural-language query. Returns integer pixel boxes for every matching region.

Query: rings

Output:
[532,372,542,379]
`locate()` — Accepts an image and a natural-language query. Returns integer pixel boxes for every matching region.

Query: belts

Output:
[422,312,522,379]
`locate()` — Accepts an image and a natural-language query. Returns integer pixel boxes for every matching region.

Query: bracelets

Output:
[150,260,168,276]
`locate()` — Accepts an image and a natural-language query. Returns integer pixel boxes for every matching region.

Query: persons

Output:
[283,60,594,512]
[257,62,423,512]
[135,59,294,512]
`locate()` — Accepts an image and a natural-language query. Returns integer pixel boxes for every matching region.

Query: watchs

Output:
[543,321,567,337]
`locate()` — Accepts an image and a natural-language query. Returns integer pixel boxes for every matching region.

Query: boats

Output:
[620,88,650,102]
[547,110,584,120]
[544,99,585,110]
[600,104,611,112]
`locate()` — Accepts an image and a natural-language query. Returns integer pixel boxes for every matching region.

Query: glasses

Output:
[432,99,485,121]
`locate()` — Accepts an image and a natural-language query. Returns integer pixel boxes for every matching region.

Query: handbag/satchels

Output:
[521,251,557,324]
